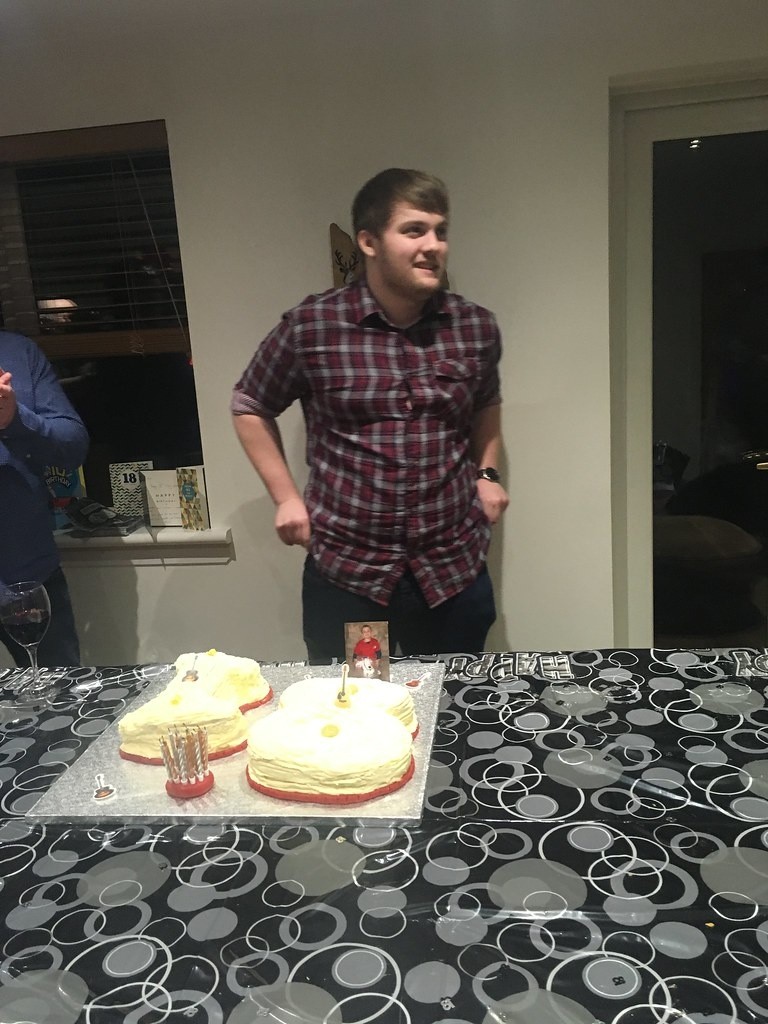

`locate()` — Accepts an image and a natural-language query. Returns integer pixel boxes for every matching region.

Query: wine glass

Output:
[0,582,61,704]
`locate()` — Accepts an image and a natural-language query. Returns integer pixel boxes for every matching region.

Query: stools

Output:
[652,514,762,632]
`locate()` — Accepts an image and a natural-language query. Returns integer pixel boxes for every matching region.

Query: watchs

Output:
[476,466,500,483]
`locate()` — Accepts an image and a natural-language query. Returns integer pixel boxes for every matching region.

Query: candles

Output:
[158,722,210,785]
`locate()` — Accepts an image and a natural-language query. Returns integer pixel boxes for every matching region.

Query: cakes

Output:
[246,705,415,804]
[118,682,248,765]
[168,649,273,714]
[279,676,419,740]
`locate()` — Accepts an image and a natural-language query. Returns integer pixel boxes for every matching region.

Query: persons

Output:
[352,624,382,667]
[0,330,92,667]
[229,164,511,663]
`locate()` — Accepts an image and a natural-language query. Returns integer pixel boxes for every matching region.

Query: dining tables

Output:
[0,645,768,1024]
